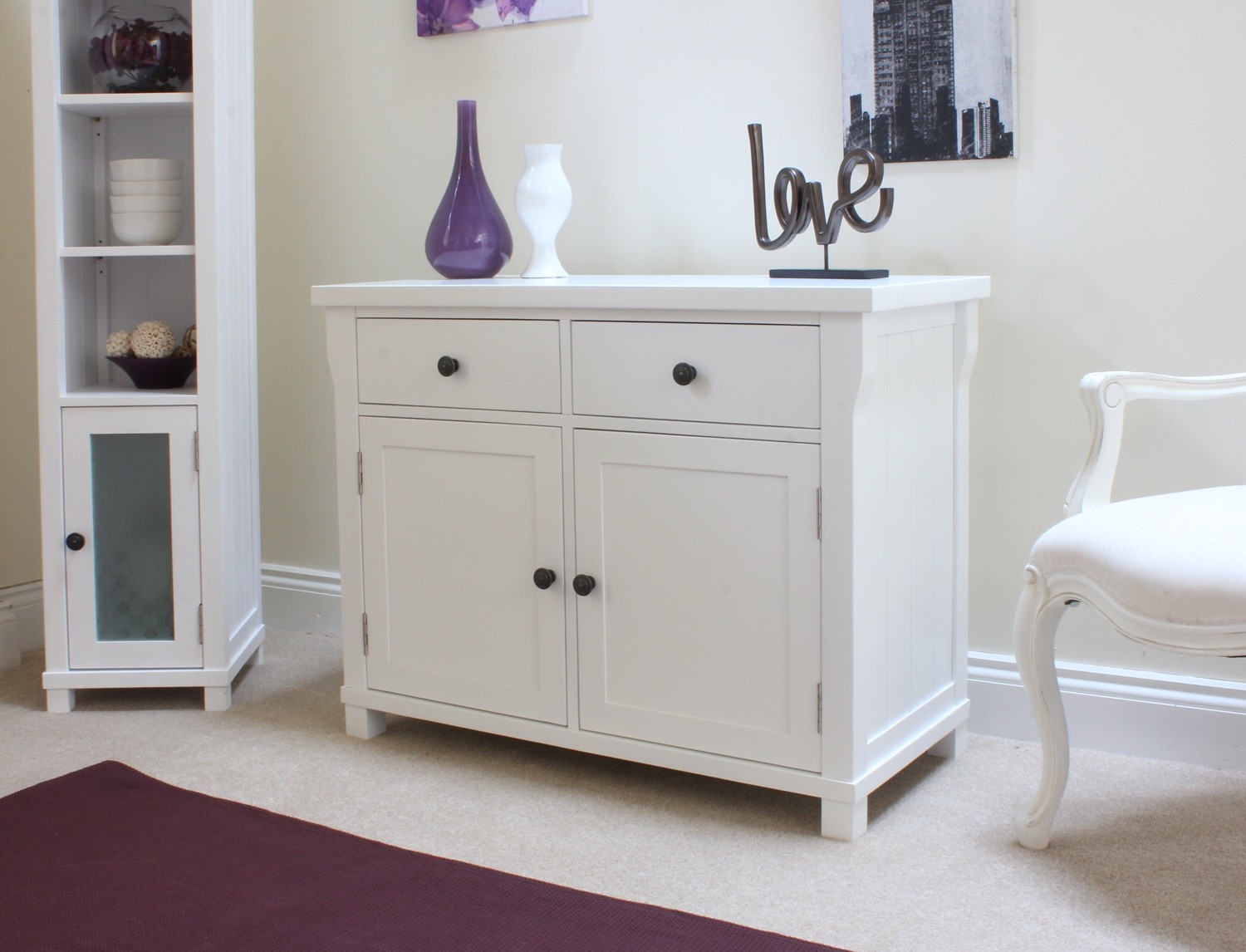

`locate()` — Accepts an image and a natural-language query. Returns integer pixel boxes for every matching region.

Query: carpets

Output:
[0,758,854,952]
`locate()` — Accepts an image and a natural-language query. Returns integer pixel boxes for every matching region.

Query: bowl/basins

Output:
[107,355,197,390]
[108,158,183,246]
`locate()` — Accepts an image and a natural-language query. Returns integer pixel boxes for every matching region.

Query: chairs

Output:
[1015,368,1246,852]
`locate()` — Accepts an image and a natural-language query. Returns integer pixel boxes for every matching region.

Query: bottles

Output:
[514,142,574,278]
[427,101,512,279]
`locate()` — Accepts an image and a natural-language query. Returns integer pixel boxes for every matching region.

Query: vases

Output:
[425,98,514,278]
[516,142,571,278]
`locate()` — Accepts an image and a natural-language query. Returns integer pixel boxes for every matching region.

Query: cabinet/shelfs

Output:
[322,274,987,835]
[32,0,265,711]
